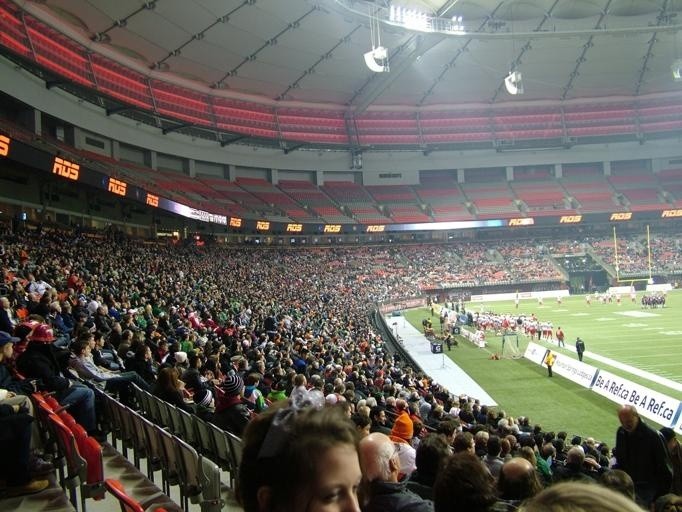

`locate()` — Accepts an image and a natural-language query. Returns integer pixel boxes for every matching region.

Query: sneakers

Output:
[30,456,55,475]
[6,480,49,496]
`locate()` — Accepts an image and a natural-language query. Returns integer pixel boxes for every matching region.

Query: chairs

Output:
[0,2,682,228]
[1,320,248,512]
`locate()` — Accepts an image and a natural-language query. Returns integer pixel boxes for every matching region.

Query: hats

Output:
[224,374,244,395]
[0,329,21,345]
[392,413,413,439]
[28,326,55,343]
[192,389,212,407]
[174,351,187,363]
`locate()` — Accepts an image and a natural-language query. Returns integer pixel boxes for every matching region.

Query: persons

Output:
[0,223,682,512]
